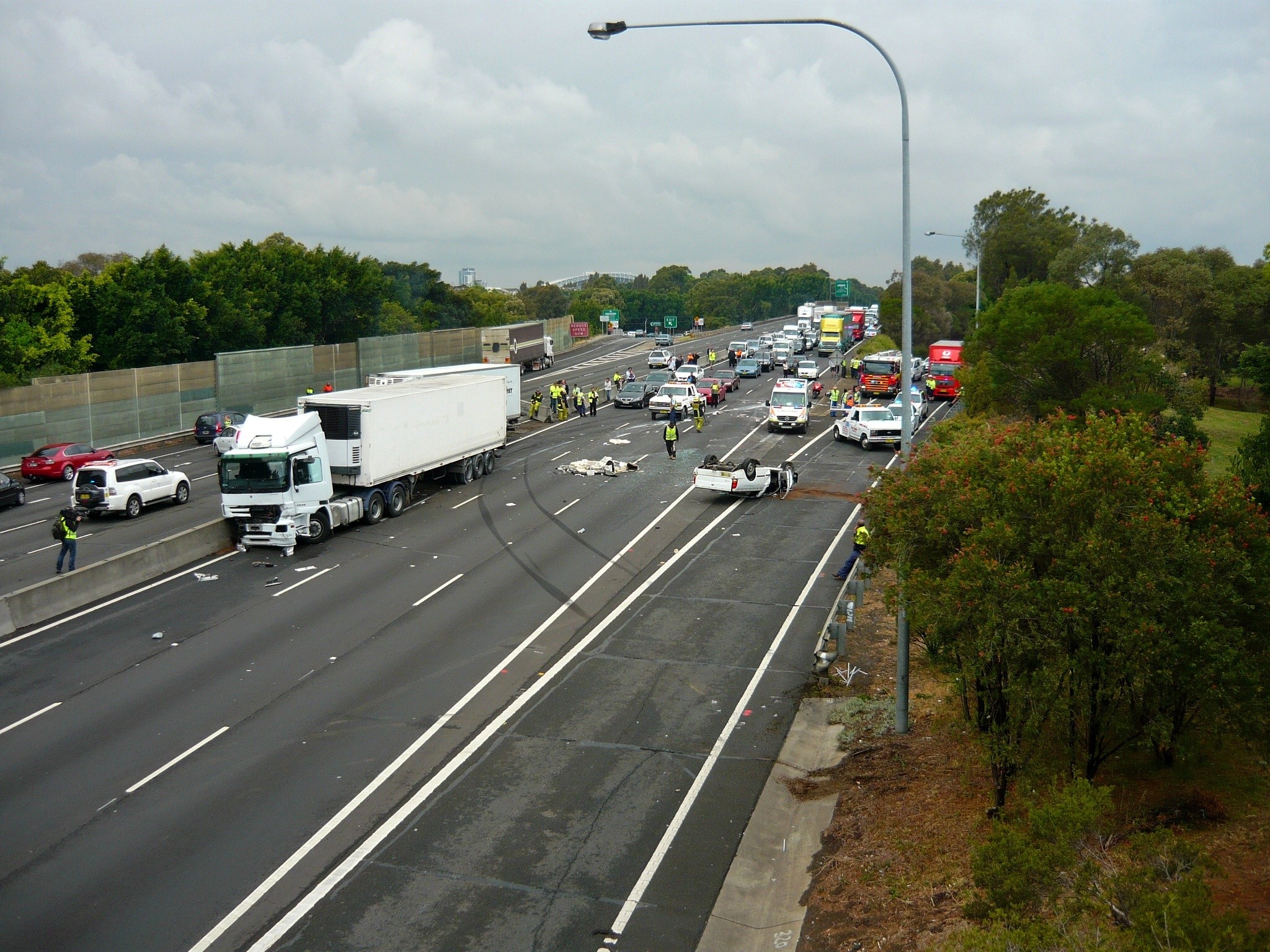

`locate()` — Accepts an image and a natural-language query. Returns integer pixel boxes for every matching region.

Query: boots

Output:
[535,411,537,416]
[529,416,532,419]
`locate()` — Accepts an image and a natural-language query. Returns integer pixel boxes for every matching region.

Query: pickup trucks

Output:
[833,405,902,452]
[648,381,707,420]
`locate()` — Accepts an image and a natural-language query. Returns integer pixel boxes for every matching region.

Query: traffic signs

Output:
[649,322,662,326]
[664,317,677,328]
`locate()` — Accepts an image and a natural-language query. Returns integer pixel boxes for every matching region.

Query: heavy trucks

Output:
[218,376,508,552]
[482,322,556,375]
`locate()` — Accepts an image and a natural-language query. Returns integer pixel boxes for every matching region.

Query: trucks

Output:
[368,363,522,430]
[765,378,812,434]
[928,340,965,399]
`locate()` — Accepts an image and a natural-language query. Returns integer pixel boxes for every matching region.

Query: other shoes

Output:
[607,401,610,402]
[669,455,672,459]
[560,420,564,421]
[56,571,61,574]
[594,415,596,416]
[564,420,568,421]
[672,457,676,460]
[834,576,846,580]
[581,415,583,417]
[696,430,702,433]
[831,573,839,577]
[591,415,593,417]
[584,415,587,416]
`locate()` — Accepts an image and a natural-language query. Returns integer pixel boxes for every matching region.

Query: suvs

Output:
[623,330,644,338]
[70,459,191,519]
[647,349,672,369]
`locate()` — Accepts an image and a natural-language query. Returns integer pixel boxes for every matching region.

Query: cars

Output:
[212,425,244,457]
[0,473,25,507]
[683,331,691,336]
[20,444,117,482]
[612,383,657,409]
[694,378,727,404]
[654,334,675,346]
[675,295,929,435]
[642,371,678,394]
[696,455,804,497]
[194,410,247,444]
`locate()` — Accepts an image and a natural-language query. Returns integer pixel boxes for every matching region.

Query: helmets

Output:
[927,374,932,378]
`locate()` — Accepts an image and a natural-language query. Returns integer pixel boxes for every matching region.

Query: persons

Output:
[710,380,720,409]
[832,519,870,580]
[707,345,715,368]
[925,375,936,401]
[799,330,807,352]
[56,506,81,574]
[728,348,741,368]
[663,422,679,460]
[306,385,314,395]
[829,356,862,385]
[323,382,333,393]
[668,352,700,372]
[691,397,704,433]
[668,393,677,425]
[529,366,635,421]
[947,387,965,406]
[687,372,697,385]
[783,358,799,378]
[221,416,233,430]
[829,387,862,417]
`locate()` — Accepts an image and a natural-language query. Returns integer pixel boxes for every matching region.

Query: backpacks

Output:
[52,516,70,540]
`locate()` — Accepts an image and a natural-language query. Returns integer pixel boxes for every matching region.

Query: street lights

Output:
[925,232,980,337]
[584,15,911,744]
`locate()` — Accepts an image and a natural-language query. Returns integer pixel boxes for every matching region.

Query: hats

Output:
[669,422,674,426]
